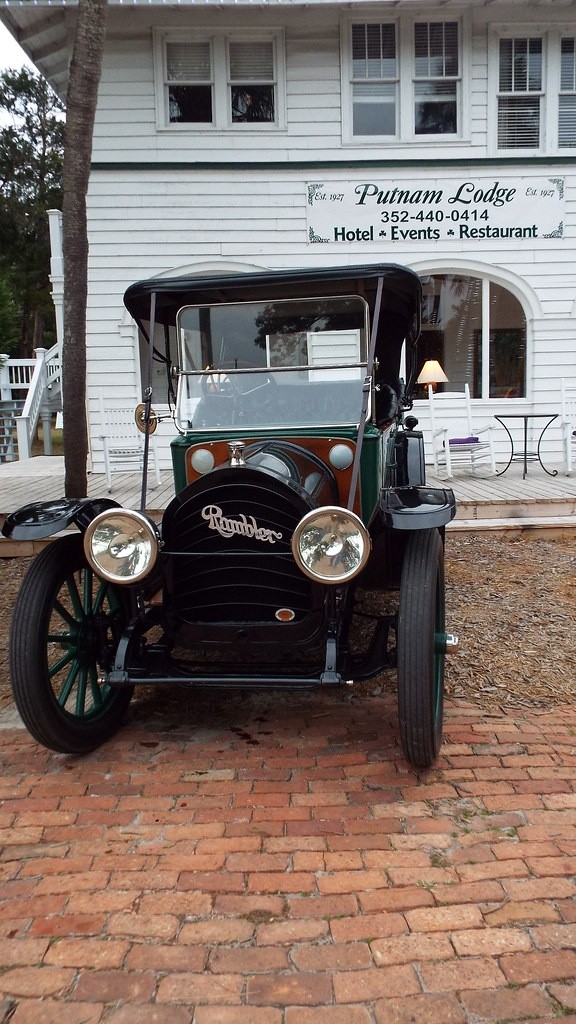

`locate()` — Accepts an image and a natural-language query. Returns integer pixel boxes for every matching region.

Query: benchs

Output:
[194,379,367,427]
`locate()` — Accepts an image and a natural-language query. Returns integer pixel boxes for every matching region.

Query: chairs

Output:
[561,378,576,475]
[429,383,497,481]
[100,393,156,492]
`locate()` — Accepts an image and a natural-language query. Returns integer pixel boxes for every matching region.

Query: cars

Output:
[1,263,457,767]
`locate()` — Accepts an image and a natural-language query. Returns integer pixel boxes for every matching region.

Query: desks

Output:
[494,413,560,478]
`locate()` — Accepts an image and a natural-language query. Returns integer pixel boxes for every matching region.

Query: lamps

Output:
[415,361,449,383]
